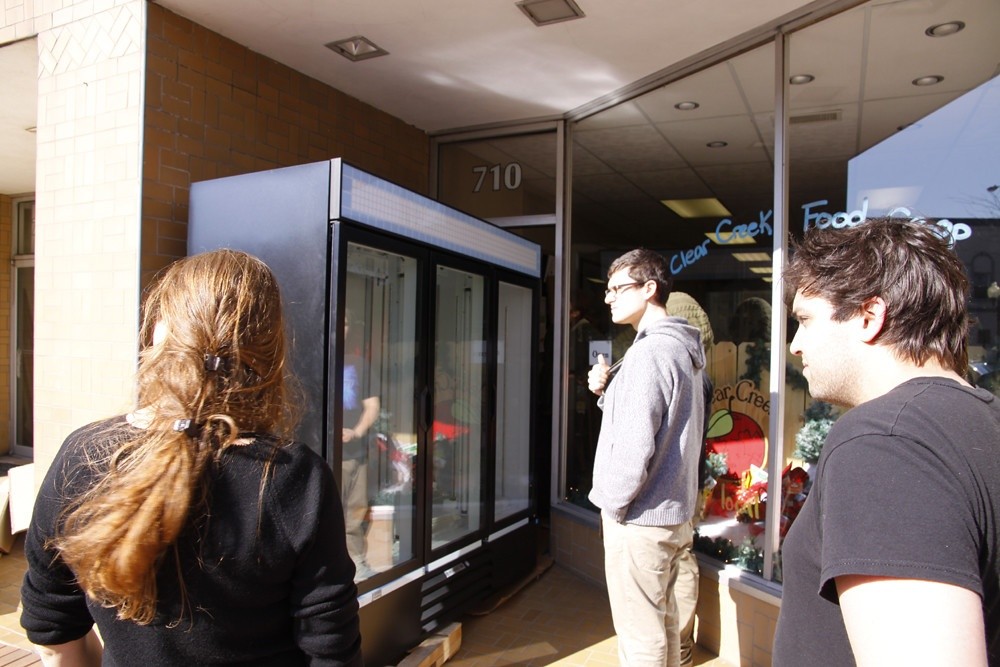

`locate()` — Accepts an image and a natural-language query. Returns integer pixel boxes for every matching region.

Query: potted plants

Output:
[696,452,728,521]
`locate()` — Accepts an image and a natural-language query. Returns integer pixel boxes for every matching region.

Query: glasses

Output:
[605,281,644,296]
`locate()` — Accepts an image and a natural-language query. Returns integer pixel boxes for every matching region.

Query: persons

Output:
[588,250,707,667]
[340,311,381,587]
[769,215,1000,667]
[20,249,365,667]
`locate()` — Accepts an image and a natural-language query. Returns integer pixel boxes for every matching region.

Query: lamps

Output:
[789,74,815,85]
[912,74,943,86]
[926,20,970,46]
[706,141,727,147]
[674,101,700,111]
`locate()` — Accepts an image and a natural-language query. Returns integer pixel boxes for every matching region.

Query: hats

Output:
[729,297,771,340]
[665,291,714,354]
